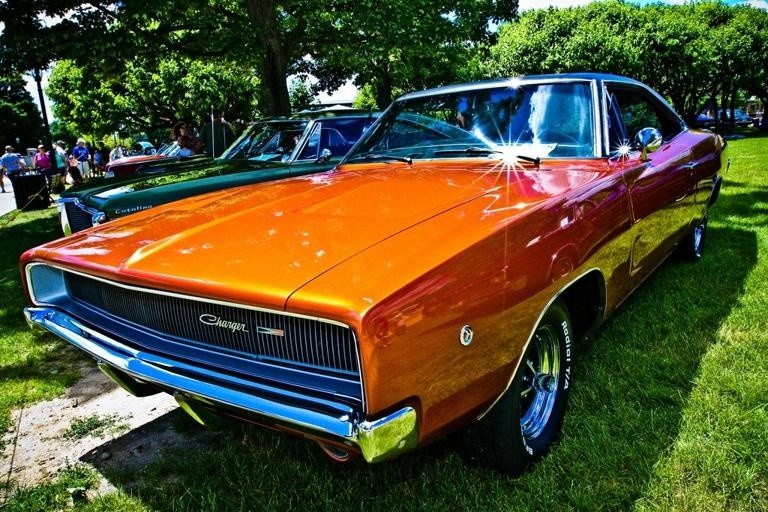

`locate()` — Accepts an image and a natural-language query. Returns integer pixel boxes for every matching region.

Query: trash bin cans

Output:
[8,168,49,210]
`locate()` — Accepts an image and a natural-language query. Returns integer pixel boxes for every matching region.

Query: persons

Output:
[0,108,236,194]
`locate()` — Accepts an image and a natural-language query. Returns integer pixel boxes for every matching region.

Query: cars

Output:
[696,109,758,131]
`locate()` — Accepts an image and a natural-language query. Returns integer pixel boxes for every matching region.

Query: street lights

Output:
[32,65,65,194]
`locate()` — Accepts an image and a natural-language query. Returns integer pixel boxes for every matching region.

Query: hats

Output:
[5,146,14,150]
[57,140,66,144]
[76,138,87,145]
[38,145,44,148]
